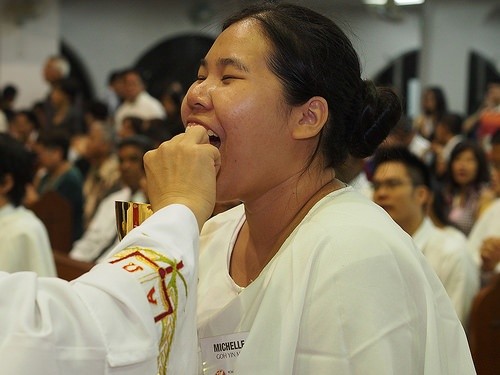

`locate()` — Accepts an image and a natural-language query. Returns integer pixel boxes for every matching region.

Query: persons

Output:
[0,0,500,375]
